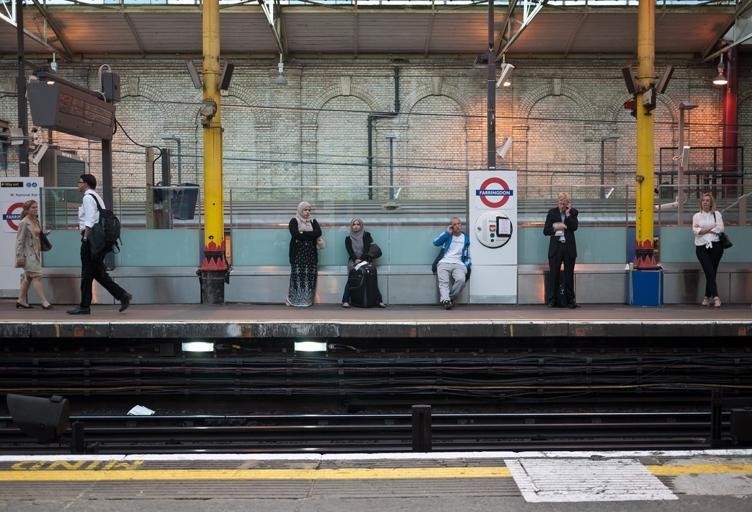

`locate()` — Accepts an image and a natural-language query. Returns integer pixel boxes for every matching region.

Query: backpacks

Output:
[86,207,123,260]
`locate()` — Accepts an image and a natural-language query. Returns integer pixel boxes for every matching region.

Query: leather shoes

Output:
[118,292,132,312]
[66,306,90,315]
[548,298,557,307]
[379,302,386,308]
[343,302,349,307]
[568,302,576,308]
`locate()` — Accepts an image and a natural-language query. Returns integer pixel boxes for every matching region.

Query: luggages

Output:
[348,261,378,308]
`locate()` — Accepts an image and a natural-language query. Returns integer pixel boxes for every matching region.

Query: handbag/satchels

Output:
[719,232,733,250]
[316,236,325,250]
[40,231,53,252]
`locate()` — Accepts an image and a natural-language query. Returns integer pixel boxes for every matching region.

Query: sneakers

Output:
[444,300,453,310]
[702,297,711,305]
[713,297,722,308]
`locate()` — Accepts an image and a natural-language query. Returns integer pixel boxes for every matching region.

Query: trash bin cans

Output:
[173,182,199,220]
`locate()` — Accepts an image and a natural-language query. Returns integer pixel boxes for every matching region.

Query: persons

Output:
[285,201,322,307]
[432,217,471,309]
[14,200,56,309]
[544,192,578,308]
[342,217,386,307]
[66,174,132,314]
[692,194,724,307]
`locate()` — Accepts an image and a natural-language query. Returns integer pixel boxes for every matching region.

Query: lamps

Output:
[274,54,287,86]
[712,52,729,86]
[499,55,511,89]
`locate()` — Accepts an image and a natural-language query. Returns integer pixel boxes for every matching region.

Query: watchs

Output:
[83,237,87,242]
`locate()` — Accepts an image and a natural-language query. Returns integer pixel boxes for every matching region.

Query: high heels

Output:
[41,304,53,310]
[16,301,34,309]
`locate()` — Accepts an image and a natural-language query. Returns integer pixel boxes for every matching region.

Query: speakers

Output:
[184,58,203,89]
[218,64,233,91]
[622,64,636,95]
[656,65,674,94]
